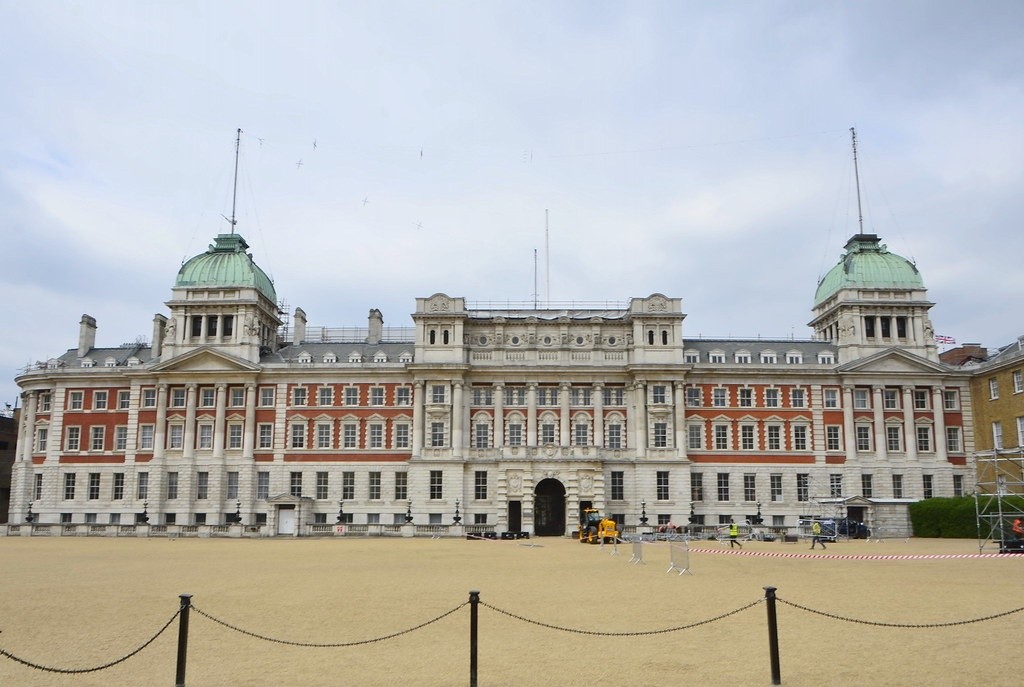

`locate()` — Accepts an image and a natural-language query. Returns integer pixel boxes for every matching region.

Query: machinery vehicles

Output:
[572,507,621,545]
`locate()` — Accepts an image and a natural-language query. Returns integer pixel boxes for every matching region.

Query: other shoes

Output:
[809,548,815,550]
[823,547,827,550]
[740,544,742,549]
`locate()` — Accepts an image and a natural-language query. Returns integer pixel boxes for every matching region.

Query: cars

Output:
[818,518,868,538]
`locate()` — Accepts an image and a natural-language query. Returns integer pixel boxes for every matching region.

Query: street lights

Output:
[756,501,763,524]
[142,500,149,522]
[25,500,34,522]
[688,500,696,523]
[639,499,648,525]
[452,498,461,523]
[336,499,345,522]
[234,500,242,523]
[404,498,413,523]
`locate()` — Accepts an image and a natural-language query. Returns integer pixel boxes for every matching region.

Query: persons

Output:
[728,519,742,549]
[809,521,826,550]
[1012,517,1024,540]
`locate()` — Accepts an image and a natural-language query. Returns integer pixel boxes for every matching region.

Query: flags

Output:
[935,335,956,344]
